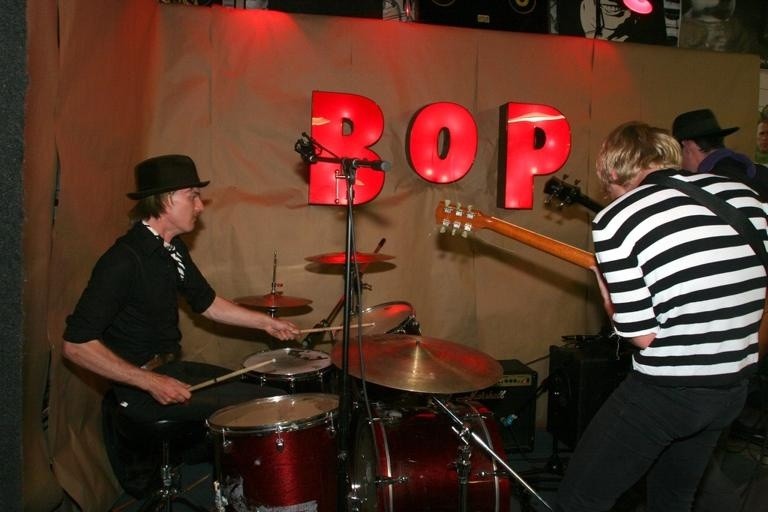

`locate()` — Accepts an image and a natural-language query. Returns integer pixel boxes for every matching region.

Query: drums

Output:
[241,347,331,393]
[357,404,512,512]
[205,392,340,512]
[339,300,421,335]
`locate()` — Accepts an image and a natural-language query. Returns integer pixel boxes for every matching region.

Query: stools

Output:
[124,416,206,512]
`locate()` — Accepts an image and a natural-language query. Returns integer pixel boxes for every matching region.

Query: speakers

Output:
[453,358,538,454]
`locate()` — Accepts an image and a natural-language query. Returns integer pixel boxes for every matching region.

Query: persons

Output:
[757,112,768,159]
[62,156,301,422]
[672,107,768,195]
[556,122,768,512]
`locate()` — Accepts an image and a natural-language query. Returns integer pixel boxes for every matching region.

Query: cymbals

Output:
[305,250,395,265]
[330,333,504,394]
[133,417,199,424]
[233,294,311,307]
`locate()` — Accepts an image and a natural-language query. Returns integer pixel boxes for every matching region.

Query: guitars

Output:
[544,174,604,213]
[435,201,597,271]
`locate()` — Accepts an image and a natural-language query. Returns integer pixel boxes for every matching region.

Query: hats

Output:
[125,154,210,200]
[671,108,741,142]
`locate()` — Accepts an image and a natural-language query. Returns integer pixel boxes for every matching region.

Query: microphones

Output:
[294,142,315,162]
[351,159,391,171]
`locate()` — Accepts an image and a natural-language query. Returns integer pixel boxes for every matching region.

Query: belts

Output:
[139,353,175,372]
